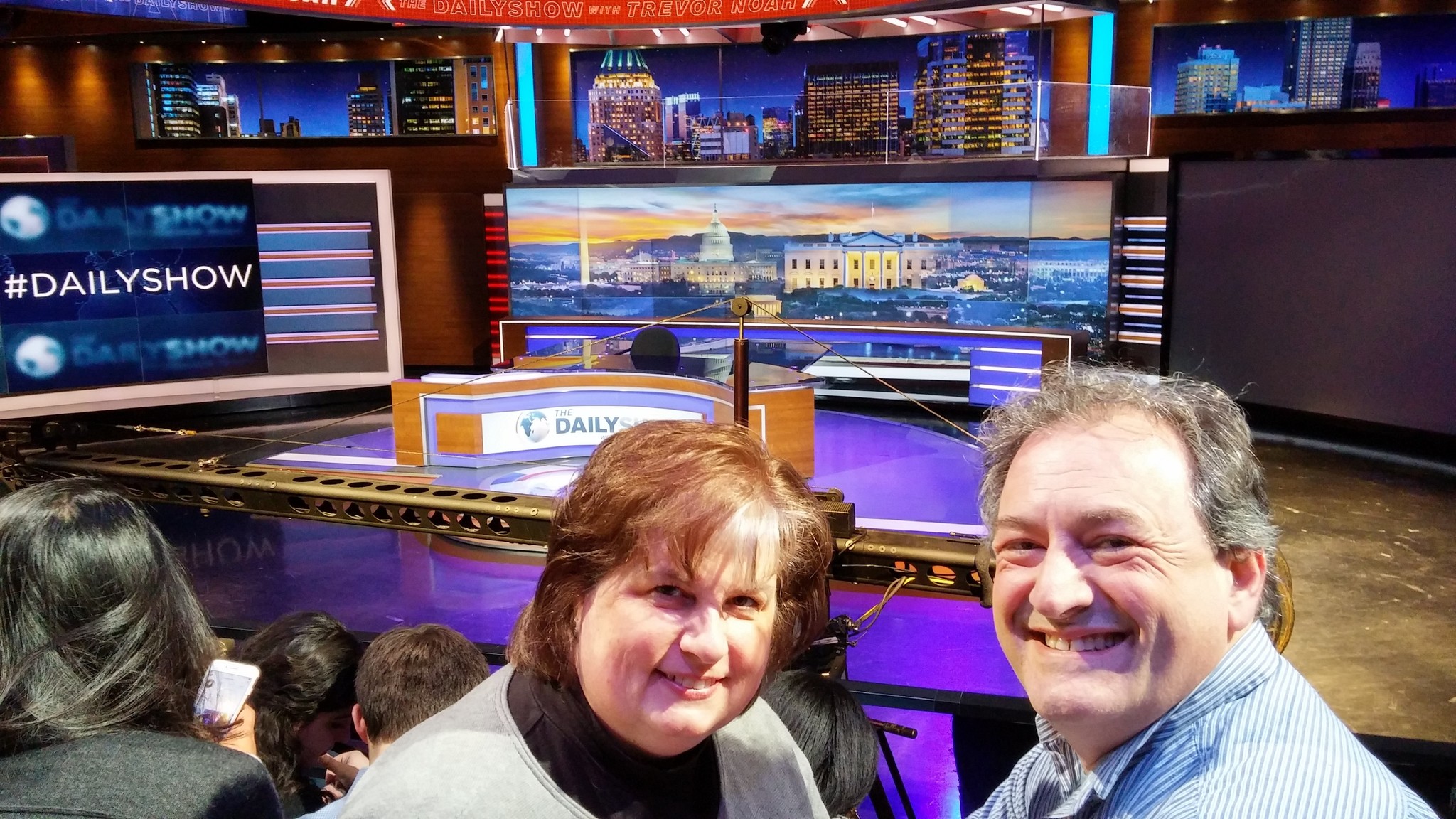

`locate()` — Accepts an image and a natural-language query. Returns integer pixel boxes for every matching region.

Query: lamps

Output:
[761,20,808,55]
[130,39,417,63]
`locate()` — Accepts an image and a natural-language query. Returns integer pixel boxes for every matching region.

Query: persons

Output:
[200,612,369,819]
[962,364,1443,819]
[755,669,878,819]
[0,478,282,819]
[293,421,830,819]
[296,624,493,819]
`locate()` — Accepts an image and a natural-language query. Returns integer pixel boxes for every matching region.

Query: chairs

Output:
[630,327,680,373]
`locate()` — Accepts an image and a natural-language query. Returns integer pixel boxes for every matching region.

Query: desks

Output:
[499,316,1087,390]
[391,355,825,480]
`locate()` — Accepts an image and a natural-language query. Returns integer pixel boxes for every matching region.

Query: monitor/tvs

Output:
[1,177,270,400]
[1158,148,1456,478]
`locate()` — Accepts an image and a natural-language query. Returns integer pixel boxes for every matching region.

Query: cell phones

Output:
[189,660,263,741]
